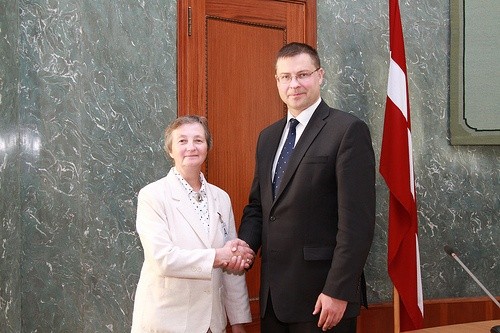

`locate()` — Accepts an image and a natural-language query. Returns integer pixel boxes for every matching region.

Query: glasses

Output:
[278,66,321,83]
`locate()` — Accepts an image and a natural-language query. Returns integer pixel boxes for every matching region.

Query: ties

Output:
[274,118,299,199]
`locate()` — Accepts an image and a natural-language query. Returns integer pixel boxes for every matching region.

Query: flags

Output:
[379,0,424,333]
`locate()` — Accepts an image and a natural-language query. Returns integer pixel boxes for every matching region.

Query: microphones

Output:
[444,245,500,333]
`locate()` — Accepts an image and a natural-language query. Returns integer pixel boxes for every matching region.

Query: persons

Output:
[223,42,376,333]
[130,114,255,333]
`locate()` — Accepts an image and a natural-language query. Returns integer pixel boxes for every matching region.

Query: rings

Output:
[246,258,250,264]
